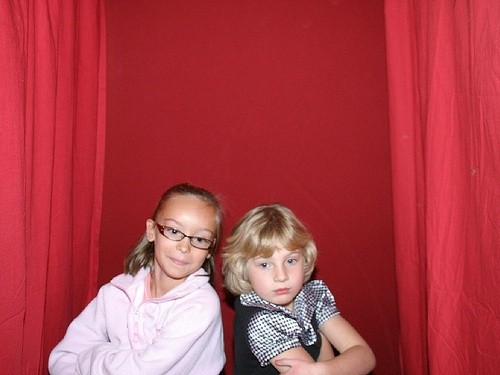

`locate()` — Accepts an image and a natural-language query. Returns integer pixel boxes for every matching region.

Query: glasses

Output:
[152,219,215,250]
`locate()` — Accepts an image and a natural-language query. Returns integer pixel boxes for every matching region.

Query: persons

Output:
[47,183,226,375]
[220,203,376,375]
